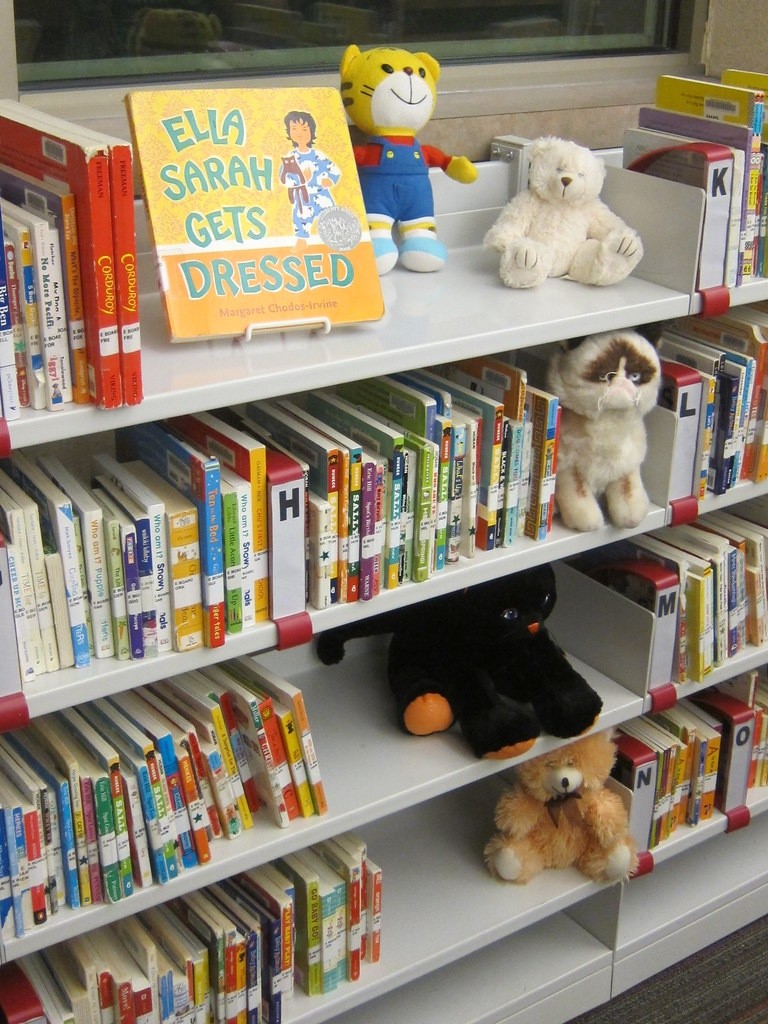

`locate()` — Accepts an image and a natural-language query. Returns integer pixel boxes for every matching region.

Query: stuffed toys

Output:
[482,725,641,891]
[481,131,647,291]
[496,329,662,533]
[336,42,477,277]
[315,563,605,762]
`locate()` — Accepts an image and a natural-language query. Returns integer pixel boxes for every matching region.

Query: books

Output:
[2,73,385,413]
[0,655,385,1023]
[621,67,768,296]
[0,355,567,695]
[655,301,768,501]
[575,509,768,855]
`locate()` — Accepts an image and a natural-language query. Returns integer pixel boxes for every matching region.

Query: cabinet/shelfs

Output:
[0,59,768,1023]
